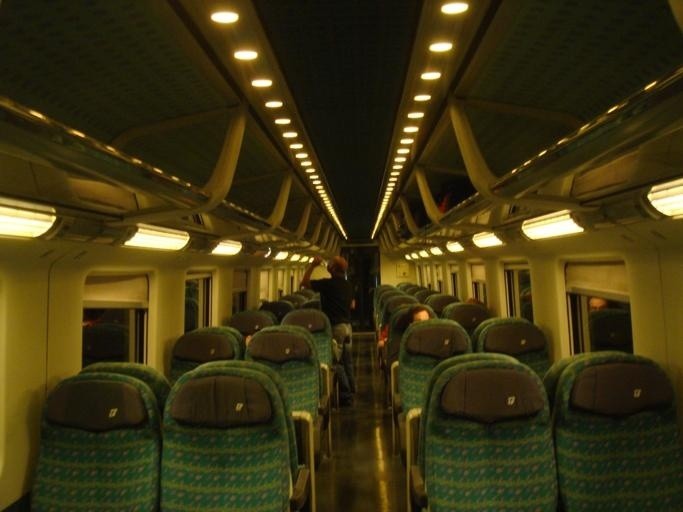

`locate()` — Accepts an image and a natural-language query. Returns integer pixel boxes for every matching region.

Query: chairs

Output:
[275,311,338,394]
[225,310,272,335]
[376,282,393,288]
[391,319,474,453]
[164,327,237,391]
[381,295,417,323]
[476,322,553,376]
[472,315,530,354]
[245,327,324,447]
[23,373,161,512]
[383,307,438,367]
[301,300,322,313]
[408,360,557,512]
[221,326,249,354]
[543,349,582,419]
[425,293,459,315]
[200,361,303,482]
[79,363,170,415]
[299,287,314,298]
[543,349,596,414]
[441,300,491,335]
[556,354,681,511]
[415,290,440,303]
[158,365,310,512]
[404,285,427,297]
[280,292,304,307]
[255,325,338,425]
[261,299,294,320]
[376,290,406,311]
[375,287,400,300]
[405,354,515,489]
[397,282,412,288]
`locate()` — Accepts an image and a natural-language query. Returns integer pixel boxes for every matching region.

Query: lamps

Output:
[206,238,243,259]
[520,207,590,242]
[639,176,683,222]
[115,222,190,252]
[469,229,510,249]
[264,247,289,261]
[0,198,57,241]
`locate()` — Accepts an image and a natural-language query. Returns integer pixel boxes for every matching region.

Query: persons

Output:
[411,306,430,322]
[300,256,356,406]
[379,324,389,347]
[588,297,607,311]
